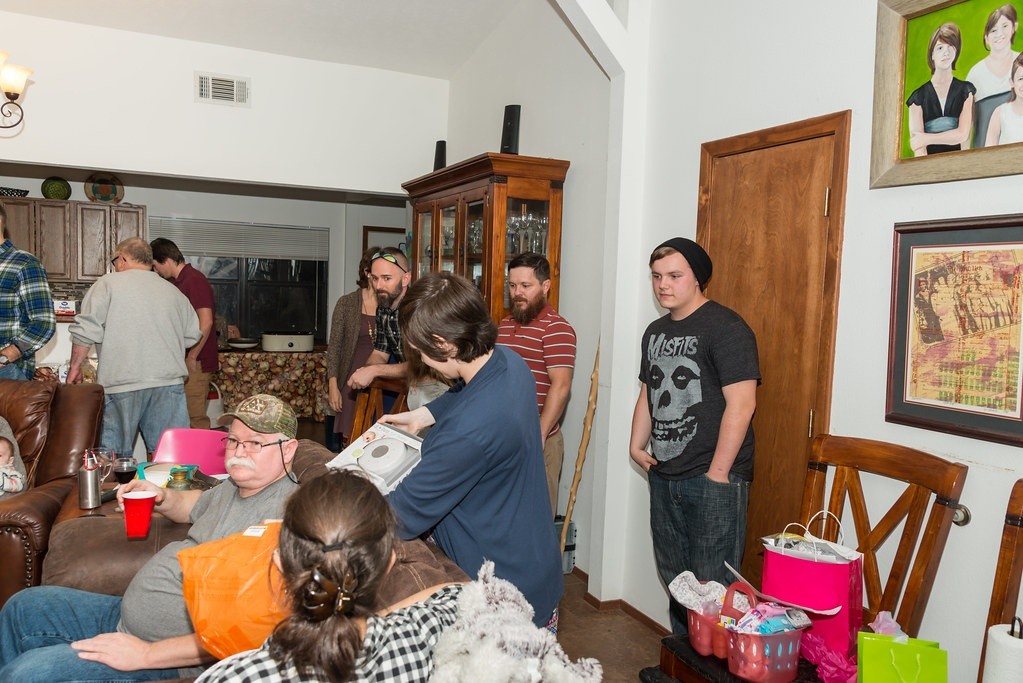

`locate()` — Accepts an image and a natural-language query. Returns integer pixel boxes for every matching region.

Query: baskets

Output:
[686,580,807,683]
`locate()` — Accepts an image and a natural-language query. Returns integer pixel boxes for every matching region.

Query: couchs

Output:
[0,379,105,610]
[41,436,604,683]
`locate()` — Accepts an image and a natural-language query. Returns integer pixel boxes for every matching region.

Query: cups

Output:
[121,491,158,541]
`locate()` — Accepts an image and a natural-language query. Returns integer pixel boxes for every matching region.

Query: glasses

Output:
[111,256,127,267]
[369,252,407,273]
[220,437,289,453]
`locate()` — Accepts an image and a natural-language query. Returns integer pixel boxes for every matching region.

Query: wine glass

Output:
[436,215,549,257]
[114,458,139,512]
[89,447,114,495]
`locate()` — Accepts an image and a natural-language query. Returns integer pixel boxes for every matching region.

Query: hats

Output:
[216,394,298,440]
[654,237,712,293]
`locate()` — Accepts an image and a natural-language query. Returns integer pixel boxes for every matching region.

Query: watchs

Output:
[0,353,10,366]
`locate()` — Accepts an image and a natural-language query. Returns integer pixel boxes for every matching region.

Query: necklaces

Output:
[362,298,377,347]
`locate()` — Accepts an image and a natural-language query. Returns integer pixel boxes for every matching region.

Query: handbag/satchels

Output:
[856,631,948,683]
[759,510,865,667]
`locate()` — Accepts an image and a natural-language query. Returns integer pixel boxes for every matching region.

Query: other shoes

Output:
[639,664,683,683]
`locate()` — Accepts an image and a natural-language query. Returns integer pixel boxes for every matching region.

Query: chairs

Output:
[348,376,415,451]
[977,477,1022,682]
[660,433,969,683]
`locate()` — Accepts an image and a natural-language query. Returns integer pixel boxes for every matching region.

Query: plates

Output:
[227,337,260,348]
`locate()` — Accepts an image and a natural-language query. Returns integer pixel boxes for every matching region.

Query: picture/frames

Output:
[883,213,1023,446]
[868,0,1023,190]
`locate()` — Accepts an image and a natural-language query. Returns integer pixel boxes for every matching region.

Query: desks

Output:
[208,349,332,423]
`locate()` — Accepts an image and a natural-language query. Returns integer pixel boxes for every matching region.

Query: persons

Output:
[628,238,760,682]
[149,238,218,430]
[0,199,57,382]
[325,248,379,453]
[348,246,452,411]
[190,469,474,683]
[0,394,302,683]
[65,237,204,482]
[0,416,28,501]
[493,250,577,522]
[373,271,565,643]
[0,436,25,497]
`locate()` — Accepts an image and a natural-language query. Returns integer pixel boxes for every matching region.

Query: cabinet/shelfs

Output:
[73,200,150,285]
[1,195,76,283]
[399,151,571,332]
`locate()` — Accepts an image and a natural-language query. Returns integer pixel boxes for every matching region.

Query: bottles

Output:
[78,450,101,509]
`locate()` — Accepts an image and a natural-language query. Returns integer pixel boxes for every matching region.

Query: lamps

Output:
[0,50,32,129]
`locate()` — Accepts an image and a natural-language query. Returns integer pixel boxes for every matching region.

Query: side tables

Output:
[52,476,162,528]
